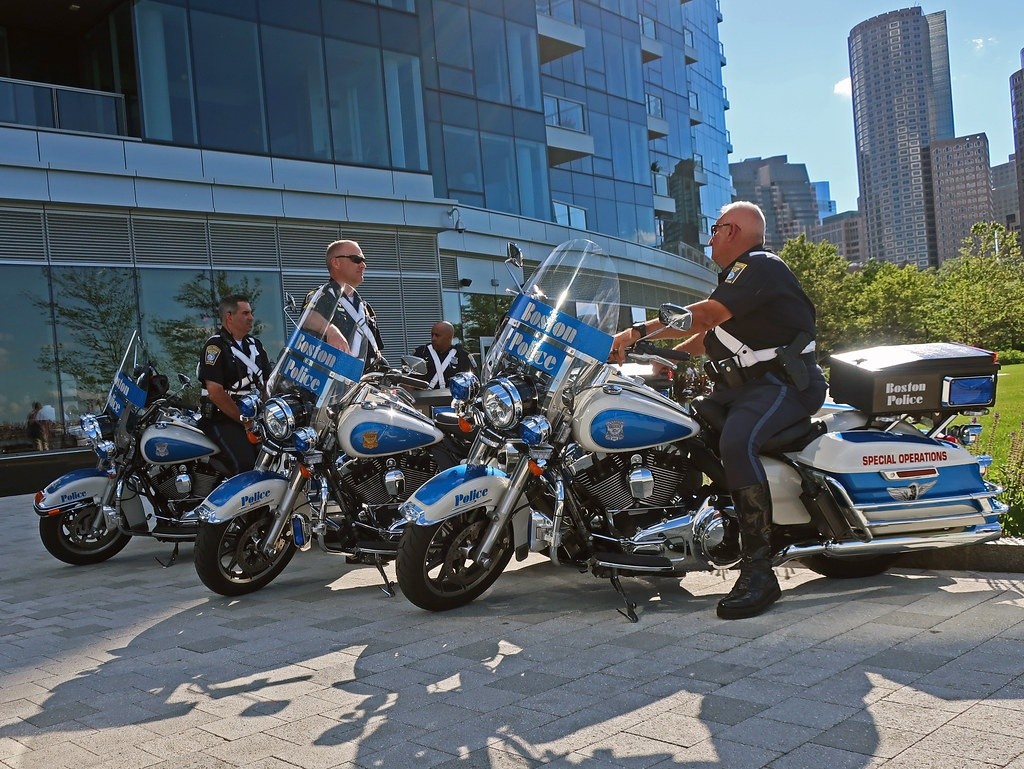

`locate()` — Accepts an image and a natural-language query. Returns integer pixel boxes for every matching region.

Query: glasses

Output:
[335,255,366,264]
[711,223,741,236]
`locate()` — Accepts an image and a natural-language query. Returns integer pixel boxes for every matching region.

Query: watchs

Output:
[632,322,647,338]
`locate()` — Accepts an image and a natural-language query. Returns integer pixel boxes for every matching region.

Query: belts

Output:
[200,388,260,397]
[704,341,816,382]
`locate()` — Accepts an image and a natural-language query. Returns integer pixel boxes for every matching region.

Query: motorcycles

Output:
[392,236,1010,624]
[30,280,689,598]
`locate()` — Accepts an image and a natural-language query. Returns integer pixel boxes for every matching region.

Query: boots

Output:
[717,480,782,620]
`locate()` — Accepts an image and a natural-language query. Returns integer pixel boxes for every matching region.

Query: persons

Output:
[198,294,274,476]
[301,240,385,372]
[27,403,56,451]
[410,321,478,389]
[607,201,828,619]
